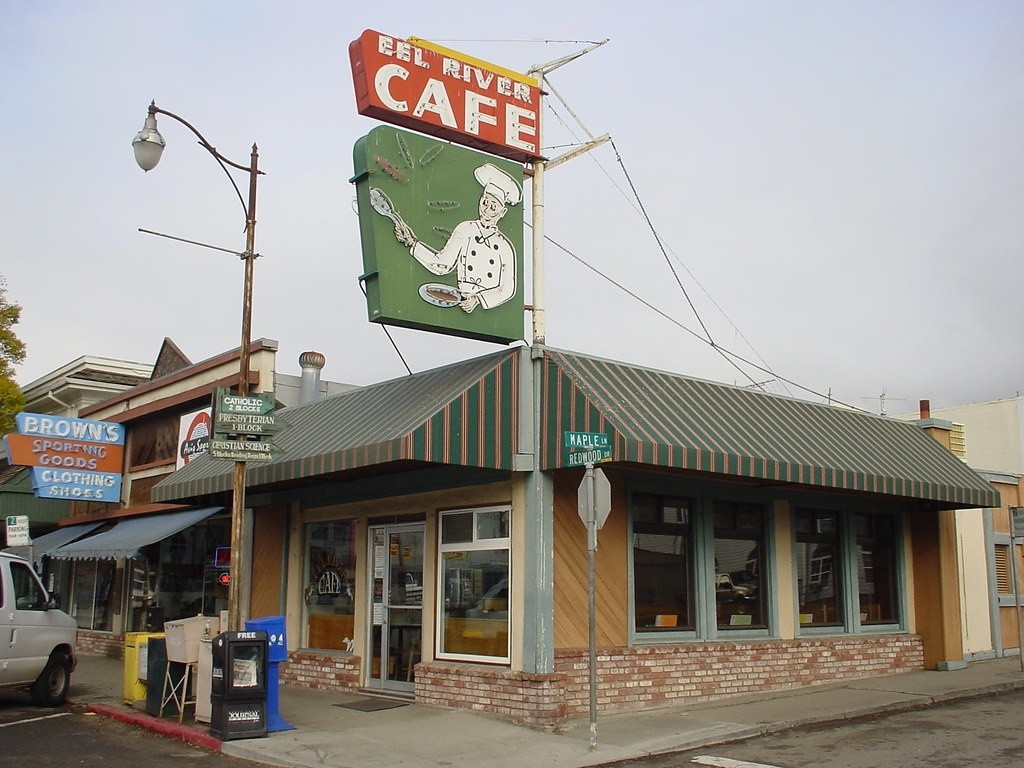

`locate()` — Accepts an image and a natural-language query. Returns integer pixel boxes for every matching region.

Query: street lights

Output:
[133,96,270,631]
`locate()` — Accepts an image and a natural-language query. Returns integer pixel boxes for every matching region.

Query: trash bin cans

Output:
[146,638,191,718]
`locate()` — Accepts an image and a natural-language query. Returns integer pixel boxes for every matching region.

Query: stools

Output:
[397,649,420,683]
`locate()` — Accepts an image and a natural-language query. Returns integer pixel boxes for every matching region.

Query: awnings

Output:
[151,345,520,509]
[1,505,224,564]
[539,348,1001,511]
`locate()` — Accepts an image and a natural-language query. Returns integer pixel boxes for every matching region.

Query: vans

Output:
[0,550,80,709]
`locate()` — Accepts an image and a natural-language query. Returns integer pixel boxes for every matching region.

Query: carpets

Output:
[332,698,410,712]
